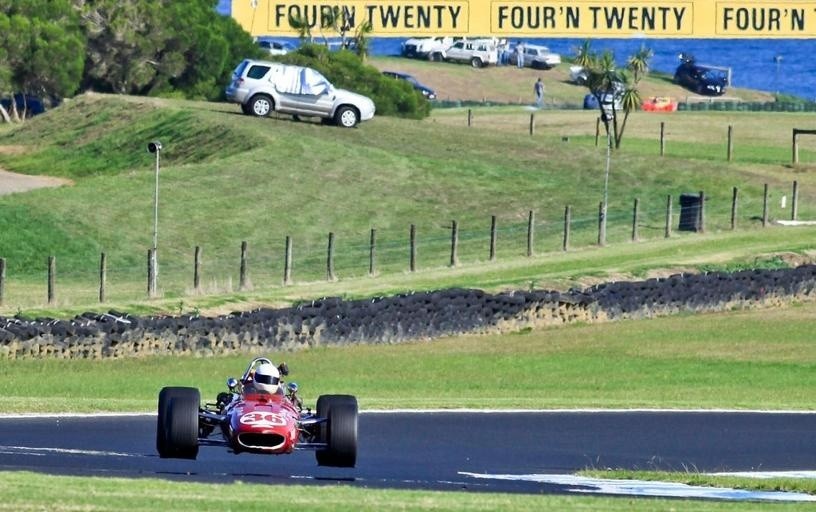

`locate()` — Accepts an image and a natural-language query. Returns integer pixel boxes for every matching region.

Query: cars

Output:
[568,66,625,110]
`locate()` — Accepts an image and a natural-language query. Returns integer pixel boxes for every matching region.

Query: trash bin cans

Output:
[678,192,704,233]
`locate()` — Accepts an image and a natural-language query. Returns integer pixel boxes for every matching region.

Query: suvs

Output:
[400,38,561,69]
[225,58,376,127]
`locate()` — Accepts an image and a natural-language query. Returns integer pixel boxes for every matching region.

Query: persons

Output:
[514,41,524,68]
[497,45,504,65]
[532,77,545,108]
[504,40,510,64]
